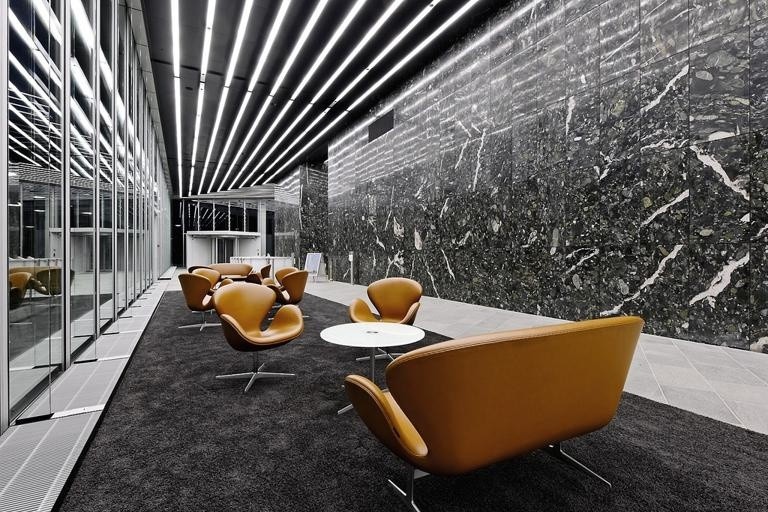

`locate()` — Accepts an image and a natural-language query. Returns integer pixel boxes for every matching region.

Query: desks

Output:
[320,321,427,416]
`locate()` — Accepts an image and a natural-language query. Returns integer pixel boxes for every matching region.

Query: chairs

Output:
[8,263,75,311]
[348,277,424,363]
[177,263,313,394]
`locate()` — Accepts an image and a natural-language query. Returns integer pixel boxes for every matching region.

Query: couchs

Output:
[344,315,645,512]
